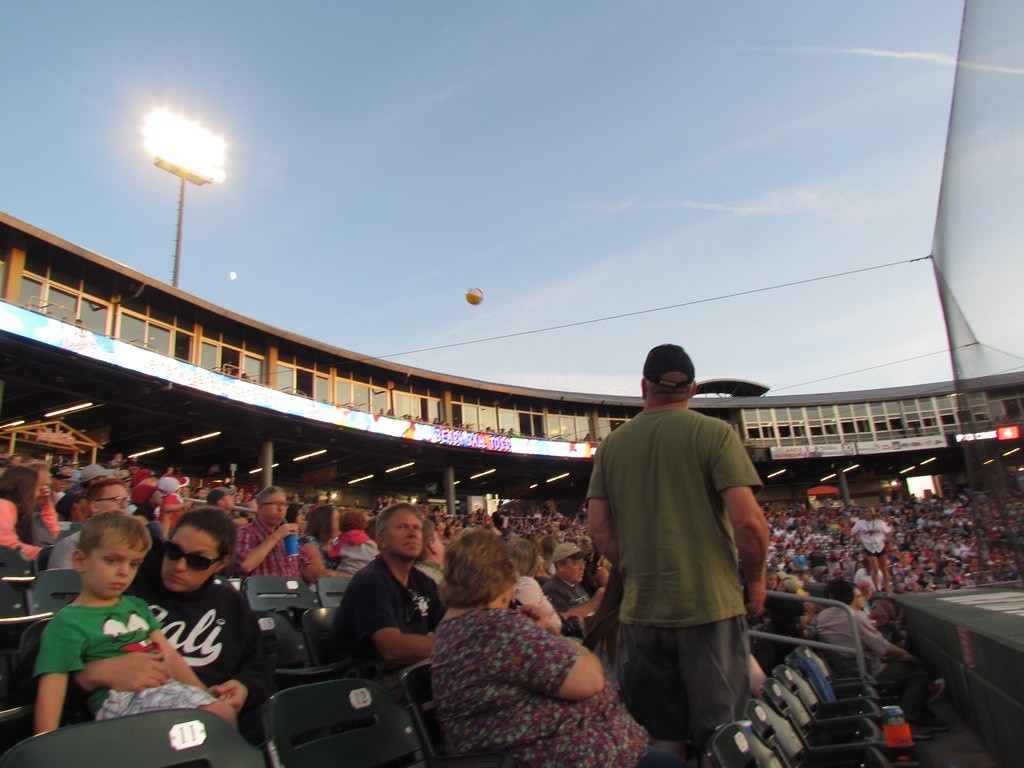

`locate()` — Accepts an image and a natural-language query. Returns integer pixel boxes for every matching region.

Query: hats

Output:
[79,464,114,481]
[777,570,800,592]
[642,343,696,391]
[553,543,592,563]
[56,492,79,513]
[158,474,190,492]
[207,487,236,504]
[48,464,70,480]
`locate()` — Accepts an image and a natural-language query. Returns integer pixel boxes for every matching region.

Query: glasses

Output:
[262,501,290,509]
[95,496,132,506]
[429,533,443,543]
[164,540,221,572]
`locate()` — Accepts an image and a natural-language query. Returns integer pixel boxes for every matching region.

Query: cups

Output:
[283,535,299,558]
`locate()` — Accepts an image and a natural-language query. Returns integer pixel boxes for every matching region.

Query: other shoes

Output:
[928,677,946,703]
[918,720,950,733]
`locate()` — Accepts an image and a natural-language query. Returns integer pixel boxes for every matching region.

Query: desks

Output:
[871,589,1024,768]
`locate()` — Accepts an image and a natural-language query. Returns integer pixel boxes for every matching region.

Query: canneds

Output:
[881,706,904,726]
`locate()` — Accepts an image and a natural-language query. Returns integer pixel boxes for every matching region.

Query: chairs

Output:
[0,513,895,768]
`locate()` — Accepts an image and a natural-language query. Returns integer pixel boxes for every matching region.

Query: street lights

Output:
[143,106,225,288]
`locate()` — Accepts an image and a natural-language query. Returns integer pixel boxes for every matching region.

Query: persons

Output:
[225,357,593,442]
[62,316,83,328]
[587,343,769,767]
[0,454,1024,768]
